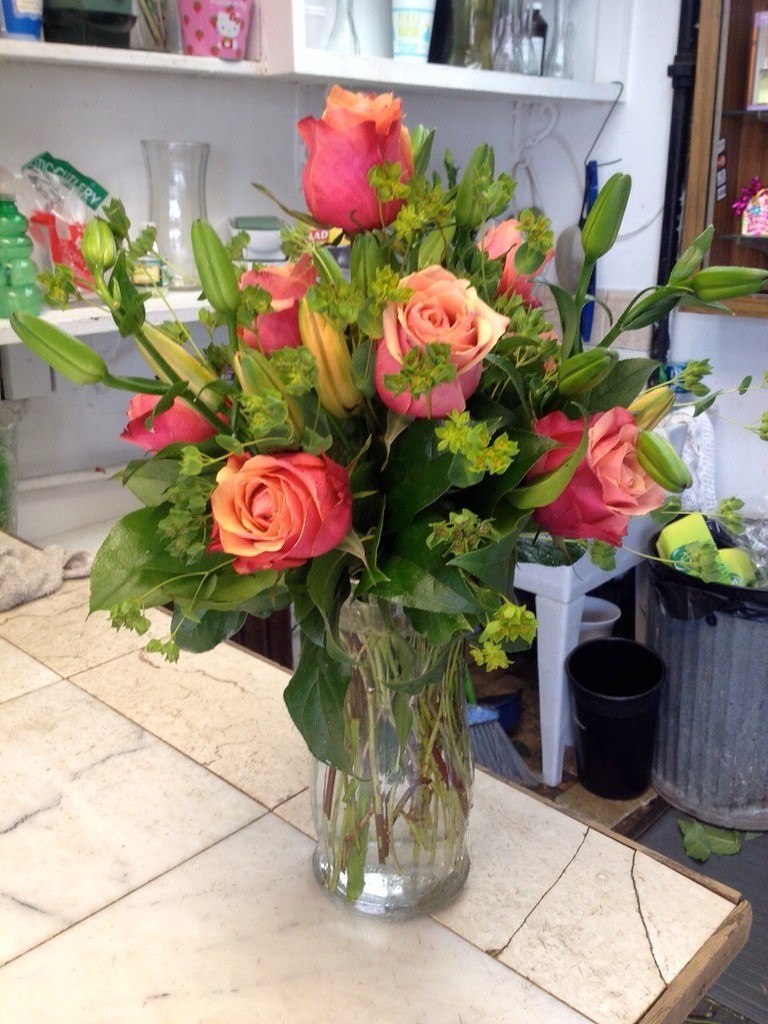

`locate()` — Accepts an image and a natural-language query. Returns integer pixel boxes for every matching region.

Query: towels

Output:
[0,530,95,613]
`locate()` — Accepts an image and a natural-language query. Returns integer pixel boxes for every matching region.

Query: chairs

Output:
[514,420,689,786]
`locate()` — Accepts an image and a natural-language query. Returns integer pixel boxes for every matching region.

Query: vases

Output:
[310,591,480,920]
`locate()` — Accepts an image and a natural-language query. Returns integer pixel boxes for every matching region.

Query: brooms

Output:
[465,656,543,789]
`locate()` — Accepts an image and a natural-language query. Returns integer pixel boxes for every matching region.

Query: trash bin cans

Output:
[564,595,622,747]
[644,515,768,833]
[564,638,666,802]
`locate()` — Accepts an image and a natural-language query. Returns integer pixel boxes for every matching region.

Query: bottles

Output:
[519,4,548,75]
[0,187,42,319]
[130,223,168,300]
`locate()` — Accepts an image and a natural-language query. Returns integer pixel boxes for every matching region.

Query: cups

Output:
[0,0,44,42]
[391,0,436,62]
[565,596,620,746]
[177,0,253,59]
[565,637,665,798]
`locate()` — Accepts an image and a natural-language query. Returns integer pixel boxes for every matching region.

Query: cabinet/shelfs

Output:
[0,0,630,353]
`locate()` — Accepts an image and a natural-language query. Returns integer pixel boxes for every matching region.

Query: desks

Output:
[0,577,752,1024]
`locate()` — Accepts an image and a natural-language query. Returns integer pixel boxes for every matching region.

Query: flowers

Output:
[8,84,729,665]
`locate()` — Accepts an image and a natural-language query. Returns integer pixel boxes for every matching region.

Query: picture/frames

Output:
[745,11,768,112]
